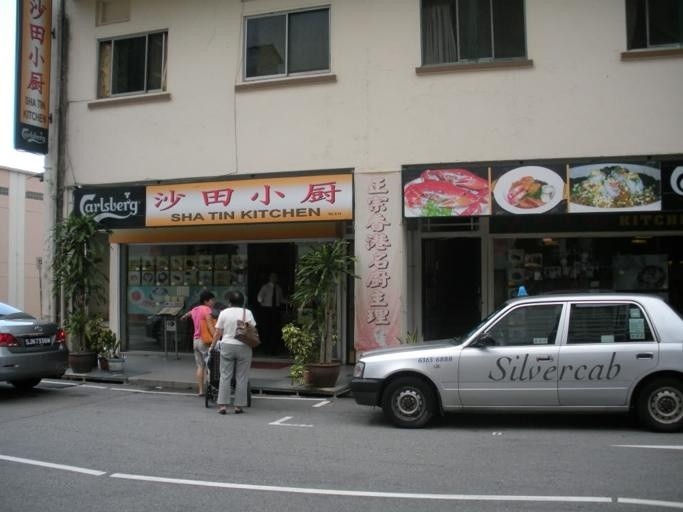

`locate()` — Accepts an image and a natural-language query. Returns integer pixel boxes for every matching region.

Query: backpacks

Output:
[234,307,262,349]
[200,306,218,345]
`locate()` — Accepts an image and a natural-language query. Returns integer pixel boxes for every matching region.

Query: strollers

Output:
[201,343,252,409]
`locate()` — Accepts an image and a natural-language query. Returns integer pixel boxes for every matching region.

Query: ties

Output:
[272,285,277,307]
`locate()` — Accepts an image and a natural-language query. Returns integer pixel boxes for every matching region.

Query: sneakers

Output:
[234,407,246,414]
[215,408,228,414]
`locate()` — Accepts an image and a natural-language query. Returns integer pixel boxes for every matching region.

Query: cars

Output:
[146,299,226,349]
[350,287,683,430]
[0,301,68,390]
[524,289,613,336]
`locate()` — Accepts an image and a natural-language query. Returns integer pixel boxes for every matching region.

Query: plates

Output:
[567,163,661,212]
[669,167,683,197]
[404,179,490,217]
[493,166,564,216]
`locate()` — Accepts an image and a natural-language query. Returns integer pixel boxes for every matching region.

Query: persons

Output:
[180,291,215,396]
[257,273,289,347]
[208,291,259,414]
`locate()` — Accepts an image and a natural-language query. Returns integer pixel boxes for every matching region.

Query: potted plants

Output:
[290,238,362,387]
[40,210,130,374]
